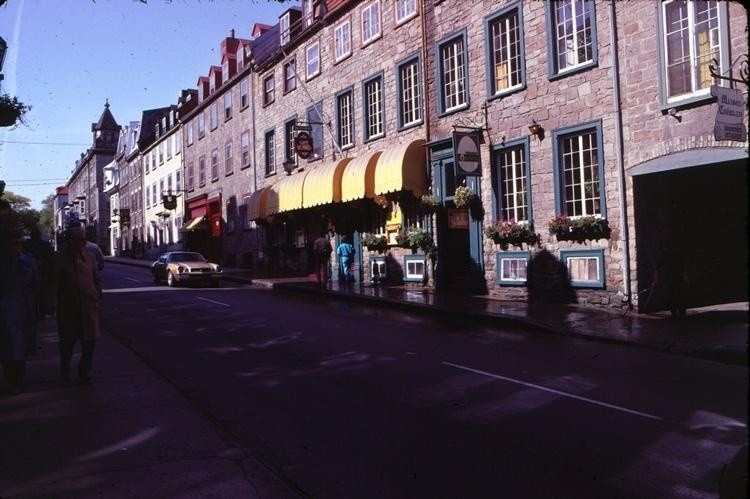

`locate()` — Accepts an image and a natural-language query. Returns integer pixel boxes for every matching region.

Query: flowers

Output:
[483,214,539,239]
[549,213,609,233]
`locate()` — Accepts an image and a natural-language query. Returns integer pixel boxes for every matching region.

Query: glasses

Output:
[10,241,26,246]
[71,236,88,243]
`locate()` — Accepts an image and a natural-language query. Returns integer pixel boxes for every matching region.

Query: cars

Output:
[151,250,225,289]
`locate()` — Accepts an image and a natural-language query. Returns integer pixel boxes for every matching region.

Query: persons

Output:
[336,234,357,284]
[312,231,333,288]
[652,239,688,322]
[1,198,105,397]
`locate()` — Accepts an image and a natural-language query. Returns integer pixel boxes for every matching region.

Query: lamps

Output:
[526,115,545,142]
